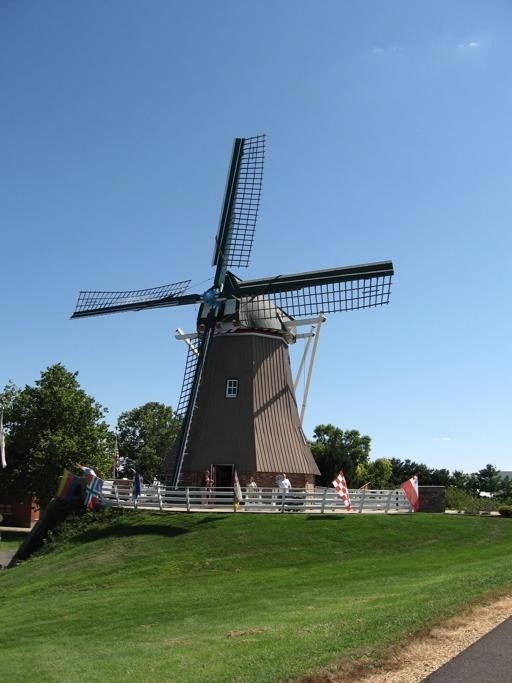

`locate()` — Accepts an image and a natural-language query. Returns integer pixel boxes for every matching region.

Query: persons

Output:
[207,479,215,505]
[275,472,292,511]
[245,476,258,504]
[199,469,211,506]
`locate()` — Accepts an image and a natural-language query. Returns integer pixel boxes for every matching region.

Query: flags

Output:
[331,470,351,511]
[400,473,420,513]
[132,469,141,507]
[233,467,244,505]
[56,463,106,510]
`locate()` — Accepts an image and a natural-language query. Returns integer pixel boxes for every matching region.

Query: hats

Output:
[248,477,255,480]
[204,471,210,474]
